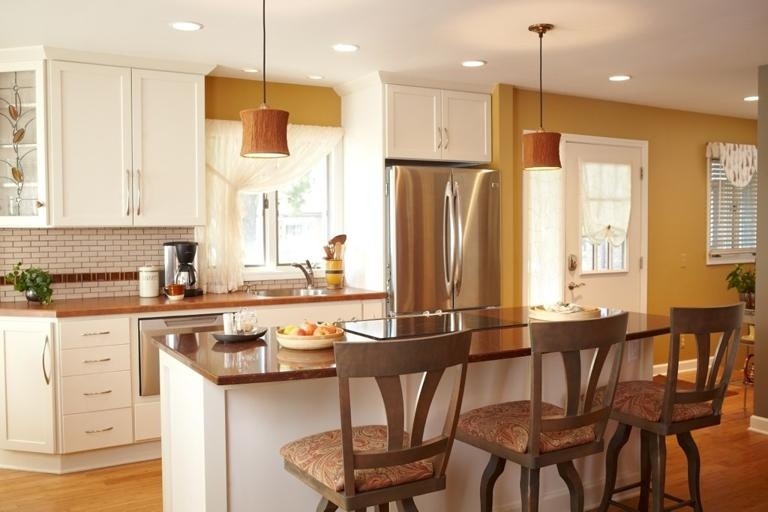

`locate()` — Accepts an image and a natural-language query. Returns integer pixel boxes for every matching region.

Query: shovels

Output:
[331,234,346,254]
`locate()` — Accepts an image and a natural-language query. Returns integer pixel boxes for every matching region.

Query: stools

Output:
[740,335,754,387]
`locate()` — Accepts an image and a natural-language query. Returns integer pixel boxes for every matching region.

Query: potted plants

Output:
[725,260,756,307]
[5,261,55,310]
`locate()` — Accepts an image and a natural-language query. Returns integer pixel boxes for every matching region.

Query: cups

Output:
[325,259,343,290]
[163,284,185,299]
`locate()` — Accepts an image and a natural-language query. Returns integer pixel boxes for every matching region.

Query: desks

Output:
[139,294,673,509]
[731,304,756,331]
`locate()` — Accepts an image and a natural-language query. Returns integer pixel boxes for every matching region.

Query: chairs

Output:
[276,332,477,512]
[448,311,633,511]
[572,303,749,509]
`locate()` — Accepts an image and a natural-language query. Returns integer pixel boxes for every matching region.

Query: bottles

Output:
[139,265,161,298]
[222,306,256,335]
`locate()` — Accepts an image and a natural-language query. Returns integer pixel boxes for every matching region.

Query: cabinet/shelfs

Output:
[56,314,135,479]
[46,50,216,227]
[0,47,47,228]
[136,397,163,463]
[1,314,58,477]
[237,300,363,326]
[334,82,495,168]
[359,297,389,319]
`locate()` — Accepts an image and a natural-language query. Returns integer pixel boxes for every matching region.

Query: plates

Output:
[211,328,267,345]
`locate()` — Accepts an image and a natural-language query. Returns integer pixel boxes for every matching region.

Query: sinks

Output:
[252,289,329,296]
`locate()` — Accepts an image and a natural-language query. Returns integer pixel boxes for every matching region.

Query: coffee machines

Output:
[162,242,204,296]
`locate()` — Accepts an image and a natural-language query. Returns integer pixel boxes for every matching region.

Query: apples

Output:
[284,321,337,336]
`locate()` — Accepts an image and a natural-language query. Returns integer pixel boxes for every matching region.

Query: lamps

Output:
[237,1,290,161]
[518,21,565,173]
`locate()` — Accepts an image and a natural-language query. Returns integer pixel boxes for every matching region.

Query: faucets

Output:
[292,260,315,288]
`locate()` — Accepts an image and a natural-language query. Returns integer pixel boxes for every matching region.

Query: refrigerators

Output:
[387,165,501,314]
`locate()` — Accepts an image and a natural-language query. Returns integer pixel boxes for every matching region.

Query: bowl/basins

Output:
[275,325,345,351]
